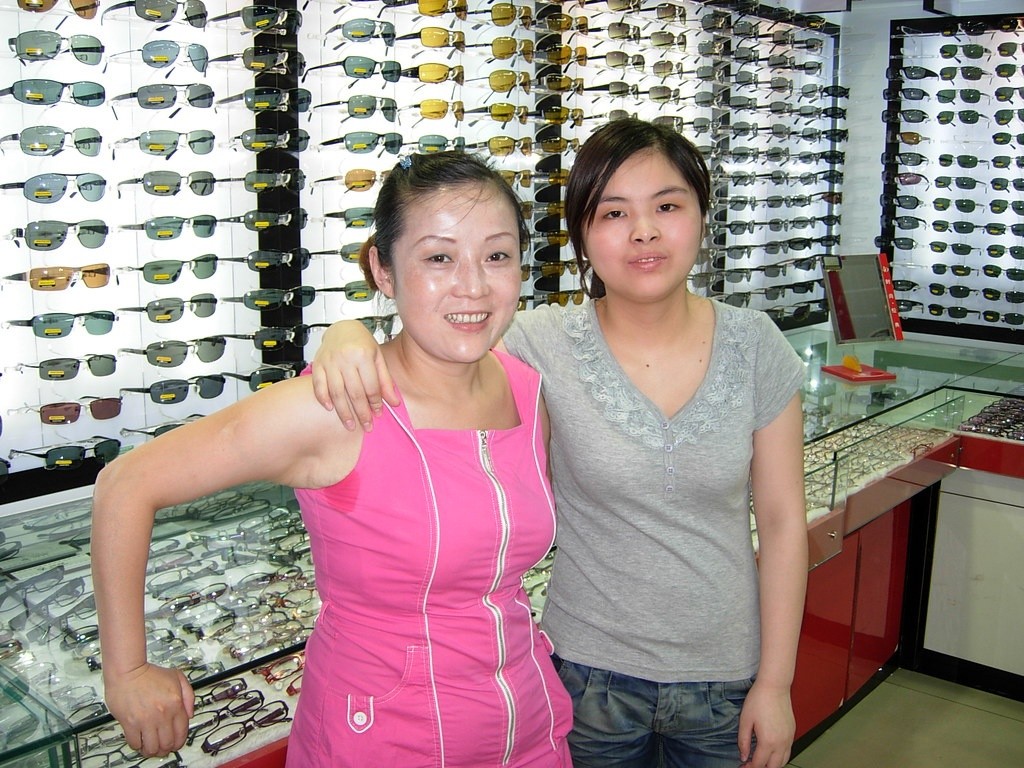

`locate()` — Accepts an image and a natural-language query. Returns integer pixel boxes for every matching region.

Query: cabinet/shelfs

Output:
[764,330,930,761]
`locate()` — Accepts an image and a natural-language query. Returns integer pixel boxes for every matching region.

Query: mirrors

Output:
[819,253,905,344]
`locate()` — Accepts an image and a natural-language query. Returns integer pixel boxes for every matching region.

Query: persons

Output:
[93,147,586,768]
[314,117,811,767]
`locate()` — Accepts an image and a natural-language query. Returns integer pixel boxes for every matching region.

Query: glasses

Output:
[0,1,1024,768]
